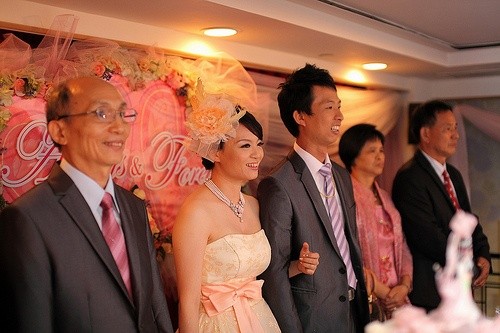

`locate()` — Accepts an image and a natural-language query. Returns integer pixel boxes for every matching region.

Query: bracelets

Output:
[401,283,409,291]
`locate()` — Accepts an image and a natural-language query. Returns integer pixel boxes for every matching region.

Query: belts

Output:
[349,288,355,302]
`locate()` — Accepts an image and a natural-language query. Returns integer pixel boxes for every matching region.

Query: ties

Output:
[100,191,133,301]
[443,169,461,212]
[318,163,358,291]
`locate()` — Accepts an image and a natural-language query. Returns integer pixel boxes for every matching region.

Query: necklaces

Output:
[320,173,336,198]
[204,178,245,223]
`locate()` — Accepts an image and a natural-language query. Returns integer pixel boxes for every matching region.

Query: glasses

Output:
[58,108,139,123]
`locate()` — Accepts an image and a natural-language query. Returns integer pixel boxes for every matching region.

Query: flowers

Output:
[0,51,199,132]
[185,94,247,145]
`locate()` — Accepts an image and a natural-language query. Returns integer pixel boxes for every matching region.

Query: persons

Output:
[257,63,371,333]
[390,98,494,315]
[0,74,176,333]
[172,78,320,333]
[338,123,413,323]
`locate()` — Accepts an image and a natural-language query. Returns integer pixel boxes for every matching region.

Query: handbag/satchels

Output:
[366,270,388,322]
